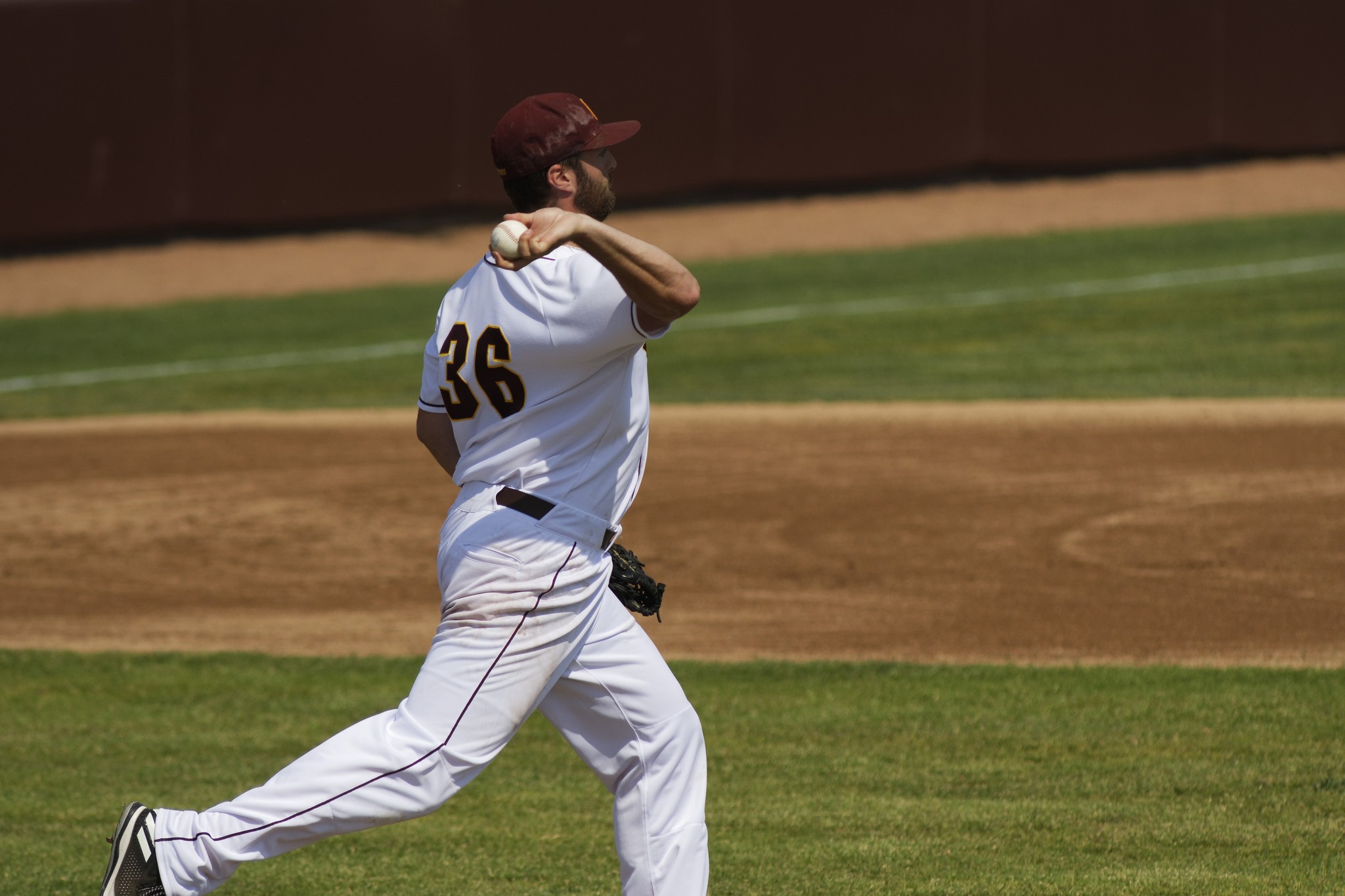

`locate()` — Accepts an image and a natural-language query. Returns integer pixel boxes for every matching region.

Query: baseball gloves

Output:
[605,543,667,619]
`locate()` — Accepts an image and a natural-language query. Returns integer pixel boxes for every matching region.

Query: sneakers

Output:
[100,802,164,896]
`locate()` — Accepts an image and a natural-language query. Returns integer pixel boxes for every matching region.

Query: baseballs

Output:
[490,220,528,259]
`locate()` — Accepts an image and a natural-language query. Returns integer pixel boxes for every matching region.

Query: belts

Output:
[493,486,618,553]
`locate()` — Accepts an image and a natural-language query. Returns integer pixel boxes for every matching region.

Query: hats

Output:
[488,90,640,174]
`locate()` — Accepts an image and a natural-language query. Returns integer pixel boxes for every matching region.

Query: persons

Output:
[98,93,709,896]
